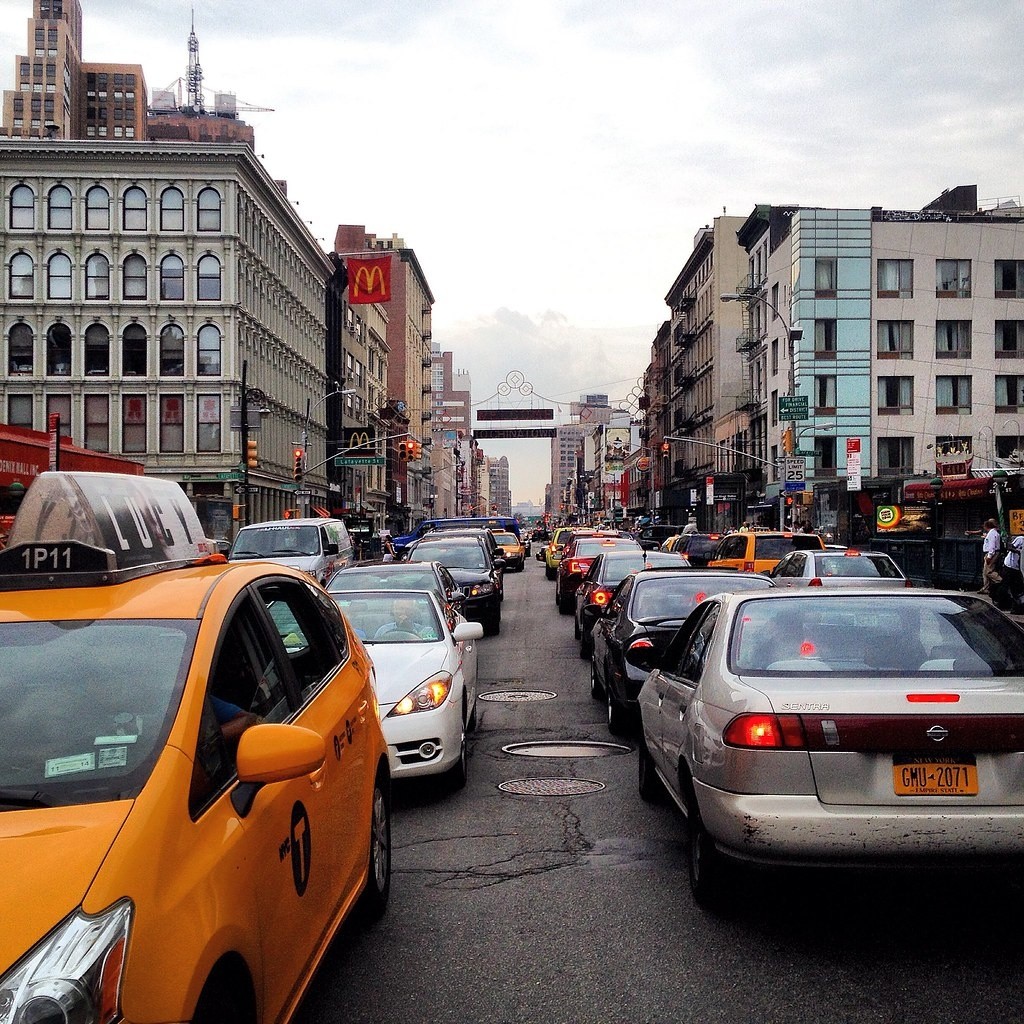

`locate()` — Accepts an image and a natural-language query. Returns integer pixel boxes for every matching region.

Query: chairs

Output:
[801,628,874,669]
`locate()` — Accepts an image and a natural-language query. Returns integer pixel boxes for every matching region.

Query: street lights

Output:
[720,292,796,533]
[299,389,359,514]
[612,437,656,522]
[240,388,273,526]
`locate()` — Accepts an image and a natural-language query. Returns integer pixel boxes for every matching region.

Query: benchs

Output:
[346,601,429,638]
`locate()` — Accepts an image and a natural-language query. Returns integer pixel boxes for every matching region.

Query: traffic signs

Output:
[234,485,263,492]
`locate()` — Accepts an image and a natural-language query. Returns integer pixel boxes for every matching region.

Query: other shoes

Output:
[977,591,989,595]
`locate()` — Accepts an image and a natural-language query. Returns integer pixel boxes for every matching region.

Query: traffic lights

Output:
[283,509,295,519]
[247,440,259,469]
[399,442,408,462]
[663,443,670,462]
[406,441,417,461]
[232,504,246,522]
[293,448,302,483]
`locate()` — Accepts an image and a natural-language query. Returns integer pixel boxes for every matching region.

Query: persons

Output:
[622,526,635,539]
[723,522,748,534]
[964,518,1004,594]
[794,520,815,534]
[382,534,395,562]
[373,598,434,638]
[1003,535,1024,614]
[538,529,549,544]
[210,695,260,745]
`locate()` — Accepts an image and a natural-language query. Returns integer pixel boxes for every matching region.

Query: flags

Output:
[347,257,391,304]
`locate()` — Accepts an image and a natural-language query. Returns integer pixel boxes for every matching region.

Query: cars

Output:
[1,471,395,1024]
[759,550,920,642]
[625,575,1024,921]
[580,568,783,736]
[266,588,484,799]
[318,523,873,661]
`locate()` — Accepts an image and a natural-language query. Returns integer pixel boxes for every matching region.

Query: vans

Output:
[390,516,521,562]
[227,517,356,587]
